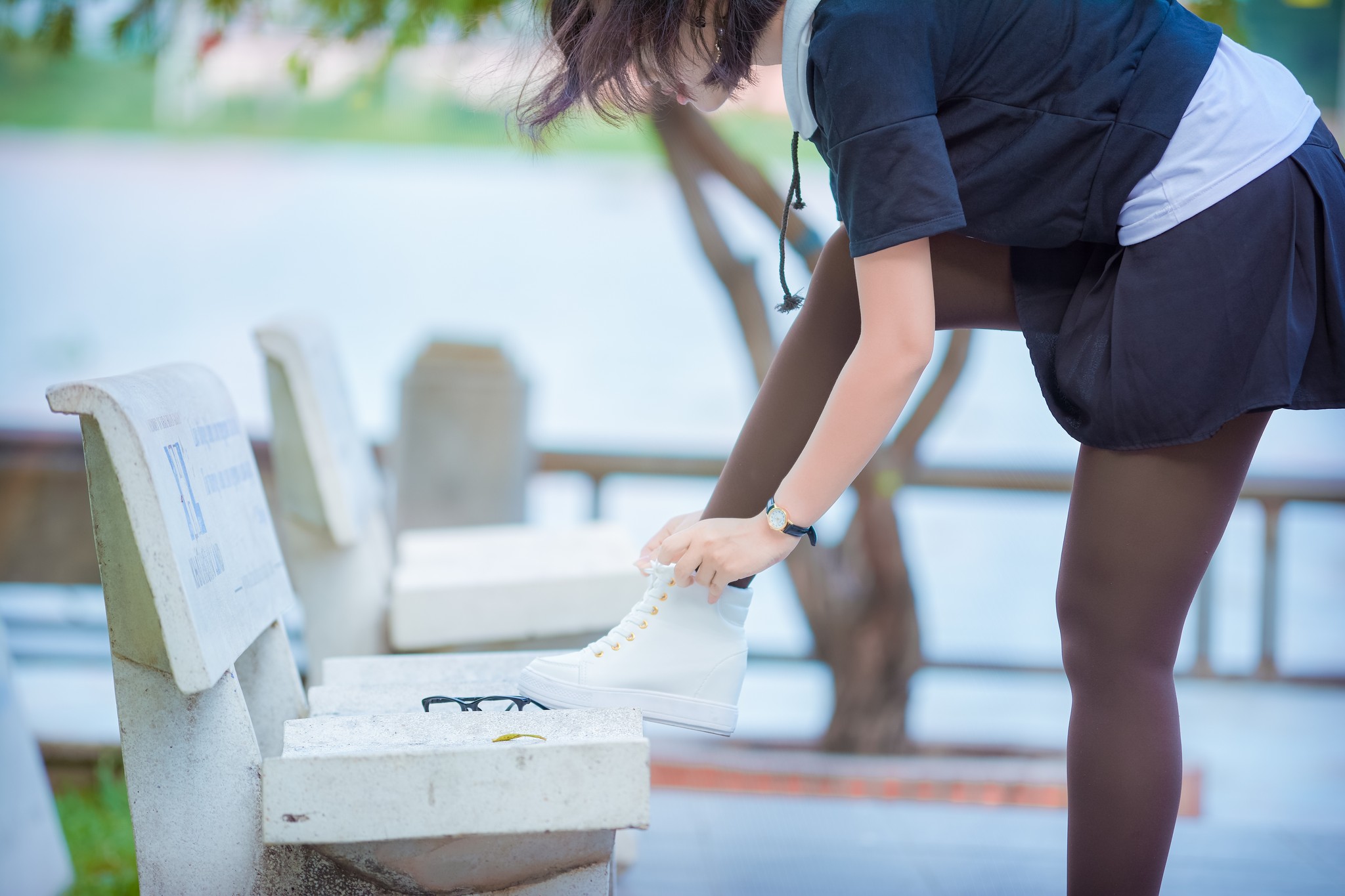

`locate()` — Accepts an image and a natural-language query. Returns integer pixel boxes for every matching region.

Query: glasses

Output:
[422,695,551,713]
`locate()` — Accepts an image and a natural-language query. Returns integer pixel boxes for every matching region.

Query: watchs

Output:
[765,498,816,548]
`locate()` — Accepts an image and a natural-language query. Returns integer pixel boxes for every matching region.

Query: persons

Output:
[516,0,1345,896]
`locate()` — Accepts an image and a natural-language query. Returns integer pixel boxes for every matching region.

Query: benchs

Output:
[43,361,654,896]
[250,314,645,688]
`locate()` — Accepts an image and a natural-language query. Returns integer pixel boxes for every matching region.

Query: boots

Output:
[521,559,755,737]
